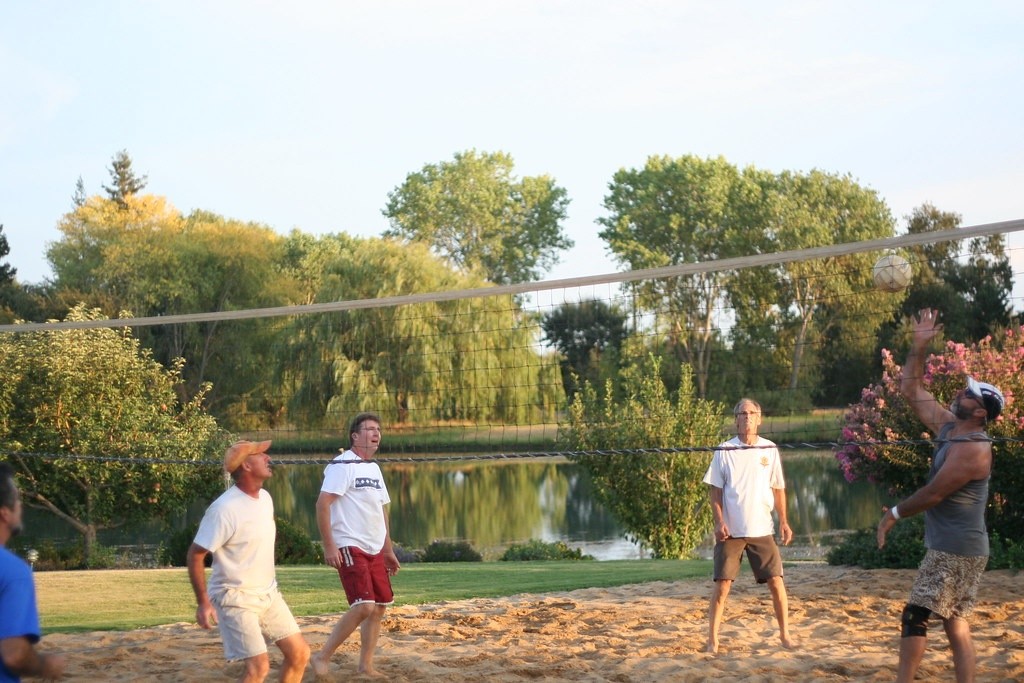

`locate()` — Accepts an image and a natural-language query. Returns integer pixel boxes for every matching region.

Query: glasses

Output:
[964,386,989,427]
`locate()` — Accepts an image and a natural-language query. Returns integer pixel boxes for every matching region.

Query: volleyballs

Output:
[873,256,912,293]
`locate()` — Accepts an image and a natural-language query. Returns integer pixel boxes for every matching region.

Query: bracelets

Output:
[892,505,900,521]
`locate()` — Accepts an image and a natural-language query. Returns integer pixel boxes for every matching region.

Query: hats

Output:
[224,440,271,474]
[967,375,1004,422]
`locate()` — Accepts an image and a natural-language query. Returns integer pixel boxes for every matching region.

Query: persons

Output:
[877,308,1005,683]
[701,399,799,653]
[0,462,64,683]
[187,440,310,683]
[310,413,400,679]
